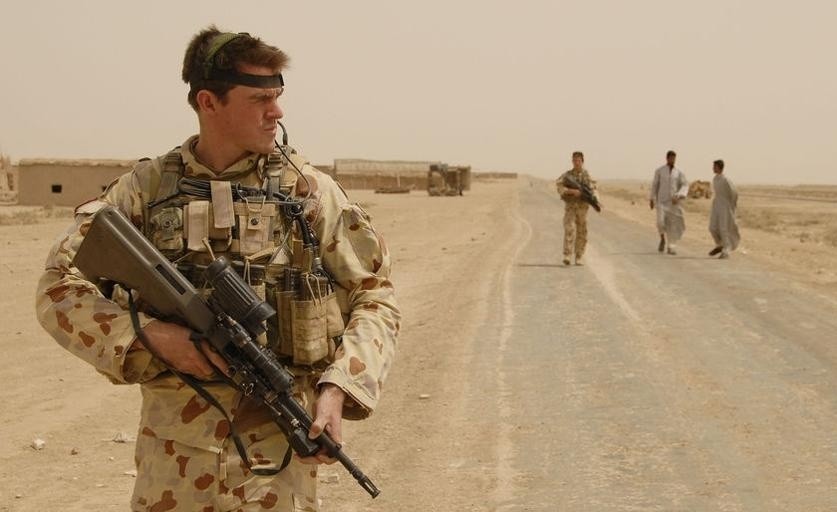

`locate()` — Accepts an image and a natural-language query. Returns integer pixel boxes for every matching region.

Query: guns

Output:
[71,205,381,499]
[564,174,601,213]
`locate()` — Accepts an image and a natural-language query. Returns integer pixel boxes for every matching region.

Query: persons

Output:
[554,151,602,266]
[32,26,404,512]
[706,158,741,260]
[648,151,690,256]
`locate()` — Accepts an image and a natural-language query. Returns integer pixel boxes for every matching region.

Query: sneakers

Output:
[575,258,583,265]
[562,257,570,265]
[718,253,729,259]
[709,246,722,255]
[658,240,665,251]
[667,247,676,254]
